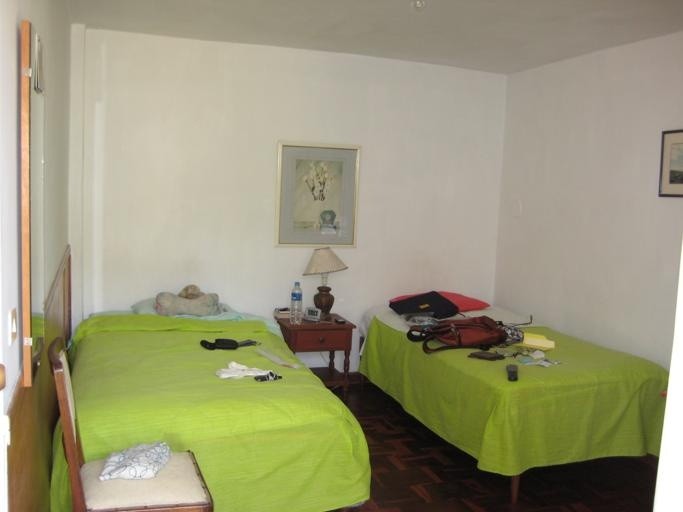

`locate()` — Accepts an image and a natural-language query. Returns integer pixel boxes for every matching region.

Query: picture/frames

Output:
[275,141,360,249]
[659,130,683,197]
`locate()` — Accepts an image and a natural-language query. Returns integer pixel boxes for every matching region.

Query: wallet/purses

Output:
[471,352,502,360]
[216,338,237,350]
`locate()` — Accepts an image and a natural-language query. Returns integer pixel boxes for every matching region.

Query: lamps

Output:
[302,247,349,322]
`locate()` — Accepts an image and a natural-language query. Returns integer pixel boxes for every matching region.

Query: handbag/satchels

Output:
[390,291,459,319]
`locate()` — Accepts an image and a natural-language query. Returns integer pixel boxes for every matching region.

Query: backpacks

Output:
[407,316,507,353]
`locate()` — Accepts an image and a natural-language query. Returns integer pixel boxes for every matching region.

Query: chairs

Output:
[48,338,213,512]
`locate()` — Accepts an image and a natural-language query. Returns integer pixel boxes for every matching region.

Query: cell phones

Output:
[335,317,345,323]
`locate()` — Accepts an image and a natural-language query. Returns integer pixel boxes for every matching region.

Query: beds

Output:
[357,304,669,504]
[50,310,371,512]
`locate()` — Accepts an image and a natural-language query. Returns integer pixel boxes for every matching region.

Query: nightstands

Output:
[275,314,356,405]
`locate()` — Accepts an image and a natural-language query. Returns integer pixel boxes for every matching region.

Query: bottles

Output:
[290,281,303,326]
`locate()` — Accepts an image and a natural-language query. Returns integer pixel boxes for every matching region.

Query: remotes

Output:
[507,364,518,380]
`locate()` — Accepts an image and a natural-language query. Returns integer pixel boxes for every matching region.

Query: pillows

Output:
[390,291,490,312]
[131,285,233,316]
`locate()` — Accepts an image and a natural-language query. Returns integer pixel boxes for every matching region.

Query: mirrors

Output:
[20,20,46,387]
